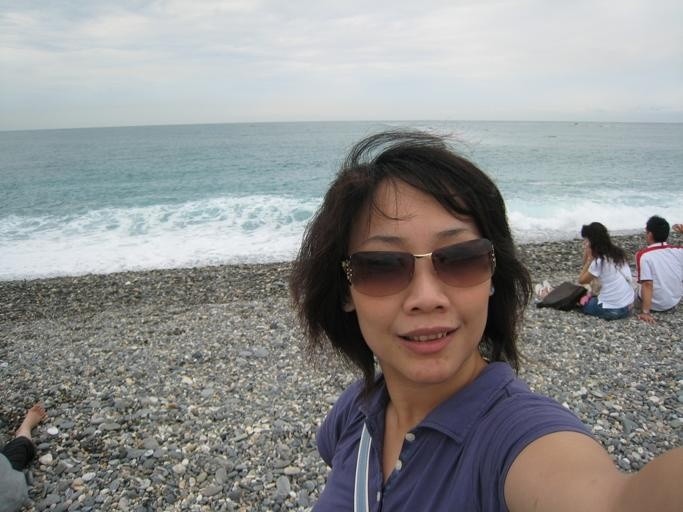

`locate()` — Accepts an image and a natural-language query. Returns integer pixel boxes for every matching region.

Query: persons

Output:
[635,215,683,325]
[287,128,683,512]
[0,403,48,512]
[535,222,635,322]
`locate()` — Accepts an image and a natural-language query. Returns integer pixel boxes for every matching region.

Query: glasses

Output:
[343,239,498,297]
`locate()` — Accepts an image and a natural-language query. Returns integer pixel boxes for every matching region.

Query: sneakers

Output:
[535,281,551,297]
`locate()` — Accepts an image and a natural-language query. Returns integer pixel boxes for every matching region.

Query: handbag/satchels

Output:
[538,282,587,312]
